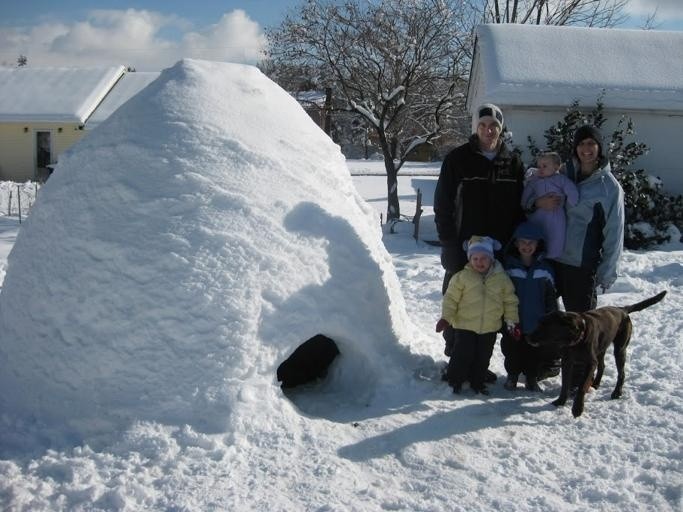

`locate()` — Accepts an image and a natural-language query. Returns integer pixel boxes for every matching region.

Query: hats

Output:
[477,102,504,130]
[463,236,501,260]
[572,125,603,156]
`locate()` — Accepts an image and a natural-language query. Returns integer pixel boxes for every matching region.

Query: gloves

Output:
[436,319,448,332]
[506,321,521,341]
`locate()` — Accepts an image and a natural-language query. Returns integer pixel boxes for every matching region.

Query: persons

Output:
[523,126,625,385]
[434,234,519,395]
[433,102,525,383]
[504,222,557,392]
[520,151,579,260]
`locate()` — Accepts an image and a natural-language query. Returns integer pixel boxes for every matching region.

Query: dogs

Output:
[524,288,668,418]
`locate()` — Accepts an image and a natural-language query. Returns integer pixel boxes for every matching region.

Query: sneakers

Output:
[537,367,560,380]
[470,382,489,394]
[453,383,461,393]
[442,375,448,381]
[484,370,498,382]
